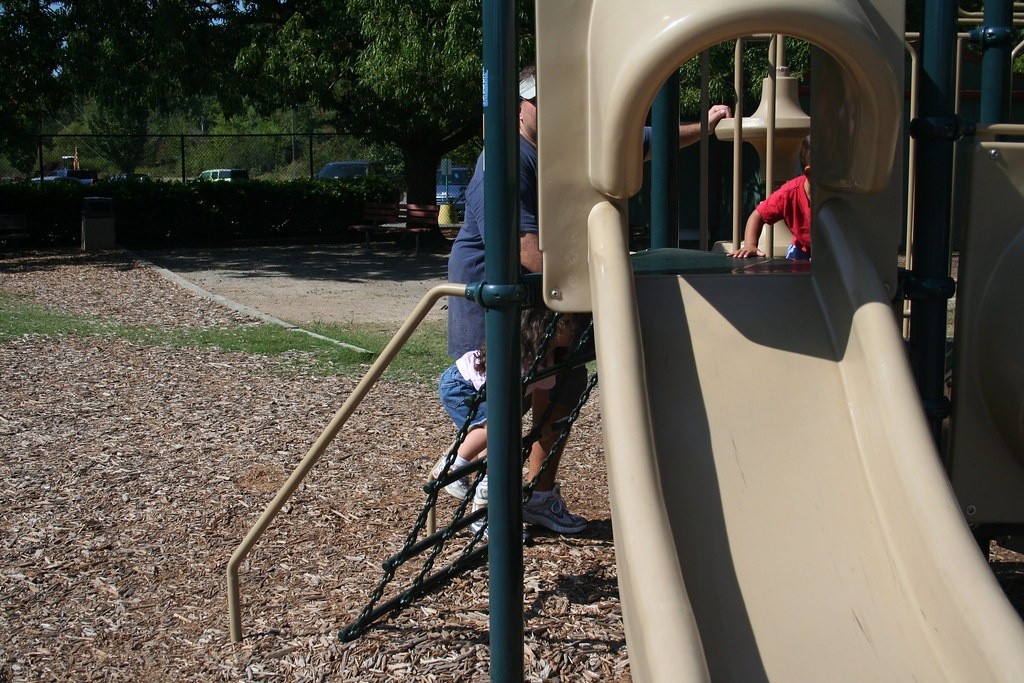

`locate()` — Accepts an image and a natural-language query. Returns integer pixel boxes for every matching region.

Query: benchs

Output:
[347,203,441,254]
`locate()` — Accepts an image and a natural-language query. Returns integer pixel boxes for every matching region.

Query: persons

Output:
[725,133,811,260]
[431,64,731,539]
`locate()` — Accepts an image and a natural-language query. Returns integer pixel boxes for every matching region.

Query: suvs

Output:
[436,164,473,219]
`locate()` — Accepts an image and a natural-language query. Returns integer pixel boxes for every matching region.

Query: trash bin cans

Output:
[82,196,116,248]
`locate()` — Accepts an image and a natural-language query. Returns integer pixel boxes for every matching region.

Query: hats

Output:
[518,75,539,101]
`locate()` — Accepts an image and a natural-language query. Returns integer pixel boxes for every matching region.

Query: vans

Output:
[197,169,249,182]
[313,162,386,180]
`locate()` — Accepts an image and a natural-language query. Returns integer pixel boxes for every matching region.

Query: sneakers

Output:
[522,482,589,532]
[432,456,471,500]
[470,487,489,538]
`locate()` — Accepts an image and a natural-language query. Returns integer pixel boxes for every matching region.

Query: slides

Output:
[587,201,1024,683]
